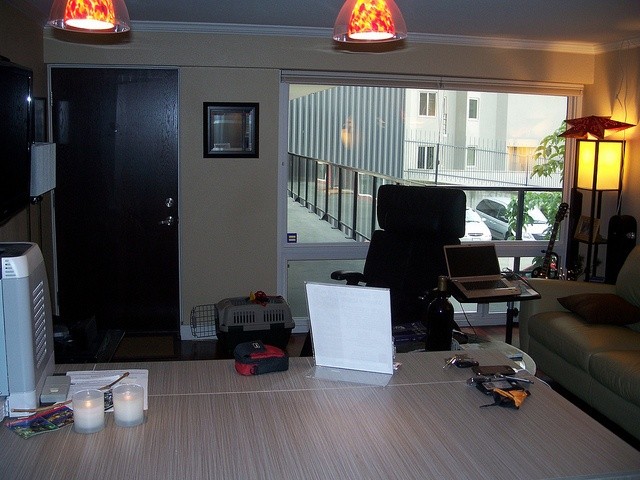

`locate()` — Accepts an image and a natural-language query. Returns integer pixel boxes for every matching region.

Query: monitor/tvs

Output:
[2,54,34,225]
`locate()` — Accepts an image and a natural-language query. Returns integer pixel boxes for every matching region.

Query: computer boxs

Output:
[425,290,454,350]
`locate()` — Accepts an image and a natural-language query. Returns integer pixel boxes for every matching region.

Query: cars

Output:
[457,206,492,241]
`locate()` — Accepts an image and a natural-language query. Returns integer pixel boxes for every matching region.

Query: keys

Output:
[442,354,475,369]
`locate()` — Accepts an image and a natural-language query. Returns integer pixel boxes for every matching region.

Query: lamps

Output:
[565,140,626,276]
[44,0,135,35]
[327,0,409,44]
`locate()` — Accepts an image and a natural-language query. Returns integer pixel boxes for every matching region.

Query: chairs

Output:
[299,186,465,357]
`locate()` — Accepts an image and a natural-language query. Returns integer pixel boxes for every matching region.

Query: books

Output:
[392,320,428,346]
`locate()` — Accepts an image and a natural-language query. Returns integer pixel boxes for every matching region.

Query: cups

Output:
[113,385,145,426]
[71,389,104,433]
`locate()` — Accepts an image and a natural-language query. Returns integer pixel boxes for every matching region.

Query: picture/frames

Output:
[202,101,259,158]
[33,98,45,144]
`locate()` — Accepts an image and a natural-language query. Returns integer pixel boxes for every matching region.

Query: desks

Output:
[448,272,542,349]
[0,348,640,478]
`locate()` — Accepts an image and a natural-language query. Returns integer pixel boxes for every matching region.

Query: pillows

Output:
[556,293,637,329]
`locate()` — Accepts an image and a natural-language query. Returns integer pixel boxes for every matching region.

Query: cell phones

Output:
[471,365,515,376]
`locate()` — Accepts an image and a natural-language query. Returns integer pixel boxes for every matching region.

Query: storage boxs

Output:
[215,296,296,349]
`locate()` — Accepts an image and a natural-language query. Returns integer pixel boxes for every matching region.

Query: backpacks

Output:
[233,343,289,376]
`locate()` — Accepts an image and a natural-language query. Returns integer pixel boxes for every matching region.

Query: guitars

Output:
[537,202,570,277]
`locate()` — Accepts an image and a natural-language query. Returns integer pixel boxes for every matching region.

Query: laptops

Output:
[443,241,521,301]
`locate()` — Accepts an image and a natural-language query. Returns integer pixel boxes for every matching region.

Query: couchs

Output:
[518,244,640,441]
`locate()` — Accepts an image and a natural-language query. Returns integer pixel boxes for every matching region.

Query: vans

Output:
[473,196,556,240]
